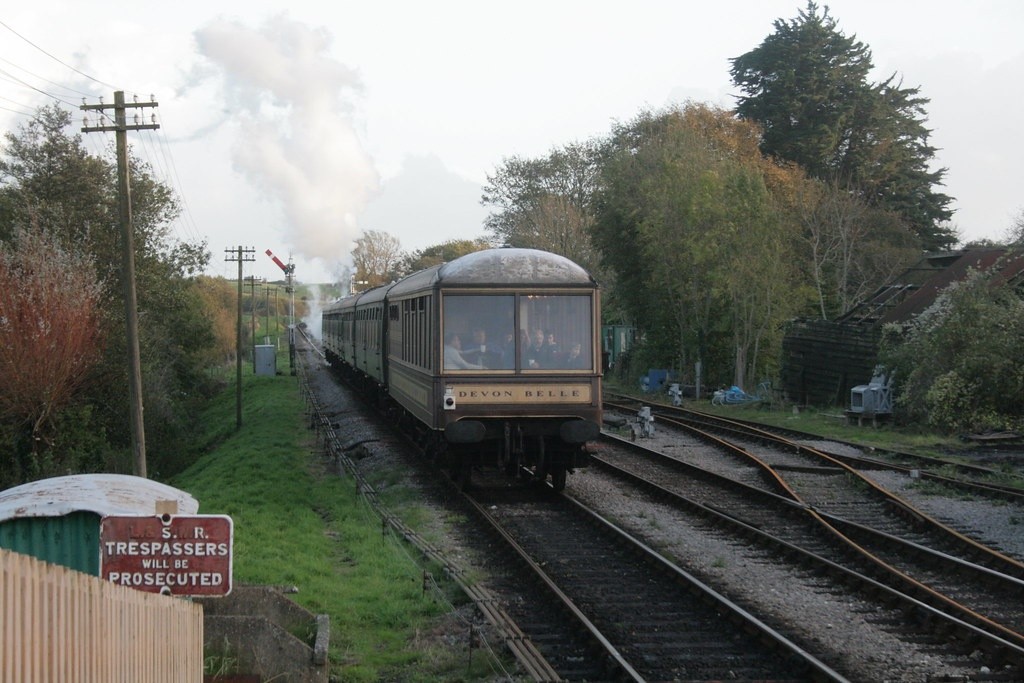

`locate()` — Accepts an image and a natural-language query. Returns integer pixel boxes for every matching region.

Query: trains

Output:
[326,246,602,490]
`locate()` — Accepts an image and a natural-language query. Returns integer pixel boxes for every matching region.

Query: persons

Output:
[442,328,584,371]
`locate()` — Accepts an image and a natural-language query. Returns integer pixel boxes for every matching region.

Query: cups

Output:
[480,344,486,352]
[528,359,535,368]
